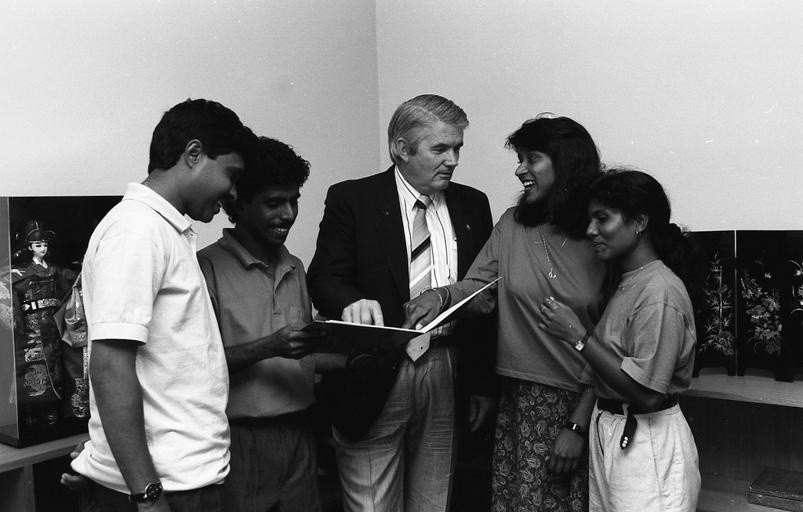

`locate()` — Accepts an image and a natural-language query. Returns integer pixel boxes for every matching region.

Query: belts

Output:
[430,333,462,349]
[592,387,690,450]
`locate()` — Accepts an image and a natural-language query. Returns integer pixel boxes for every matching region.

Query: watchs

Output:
[573,330,590,352]
[129,481,165,503]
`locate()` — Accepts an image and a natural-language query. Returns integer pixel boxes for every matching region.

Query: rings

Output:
[546,300,552,306]
[542,307,547,312]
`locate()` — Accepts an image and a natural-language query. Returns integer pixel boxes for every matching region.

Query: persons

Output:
[306,95,493,511]
[401,113,619,512]
[196,136,375,511]
[539,171,701,512]
[61,99,258,512]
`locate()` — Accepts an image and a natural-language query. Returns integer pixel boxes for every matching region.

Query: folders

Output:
[299,277,502,351]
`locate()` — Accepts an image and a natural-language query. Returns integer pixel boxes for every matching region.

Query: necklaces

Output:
[538,225,569,279]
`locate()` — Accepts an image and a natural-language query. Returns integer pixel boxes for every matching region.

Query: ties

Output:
[402,193,440,365]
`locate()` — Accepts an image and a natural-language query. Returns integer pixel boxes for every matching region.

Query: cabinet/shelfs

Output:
[680,367,801,512]
[0,422,90,512]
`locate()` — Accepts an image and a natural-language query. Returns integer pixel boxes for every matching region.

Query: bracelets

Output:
[561,421,587,435]
[420,287,443,308]
